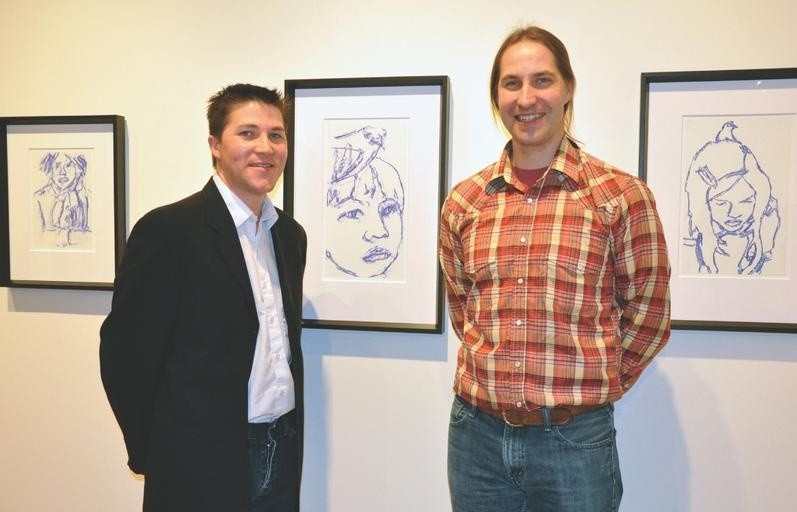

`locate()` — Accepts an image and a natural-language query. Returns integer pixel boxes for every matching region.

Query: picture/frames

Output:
[284,77,447,335]
[1,116,127,293]
[636,67,797,332]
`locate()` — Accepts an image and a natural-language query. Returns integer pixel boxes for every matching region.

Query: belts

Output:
[477,405,597,428]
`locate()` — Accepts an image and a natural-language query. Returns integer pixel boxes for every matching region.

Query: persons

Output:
[322,147,407,281]
[435,27,672,512]
[33,151,91,233]
[683,116,782,275]
[98,84,309,512]
